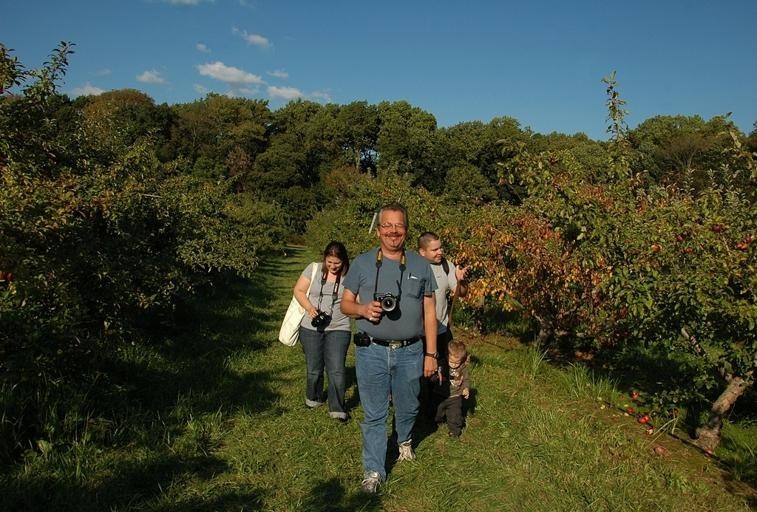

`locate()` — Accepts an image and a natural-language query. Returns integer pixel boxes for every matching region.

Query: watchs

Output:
[425,351,439,359]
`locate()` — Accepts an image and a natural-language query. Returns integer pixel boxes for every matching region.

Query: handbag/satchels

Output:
[278,261,319,347]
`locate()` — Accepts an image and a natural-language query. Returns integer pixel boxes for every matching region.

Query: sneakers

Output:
[396,437,418,462]
[305,398,324,409]
[361,468,382,493]
[328,410,348,420]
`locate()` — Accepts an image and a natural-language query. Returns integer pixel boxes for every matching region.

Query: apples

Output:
[624,216,756,454]
[0,270,15,281]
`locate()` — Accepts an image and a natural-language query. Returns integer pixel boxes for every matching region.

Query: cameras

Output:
[374,292,397,313]
[311,308,332,327]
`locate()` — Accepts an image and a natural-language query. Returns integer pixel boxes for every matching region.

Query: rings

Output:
[368,316,372,322]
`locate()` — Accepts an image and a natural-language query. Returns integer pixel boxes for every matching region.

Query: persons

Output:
[341,202,439,495]
[292,240,361,421]
[417,231,473,429]
[435,340,471,439]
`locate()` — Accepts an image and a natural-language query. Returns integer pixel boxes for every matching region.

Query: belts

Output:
[372,334,424,350]
